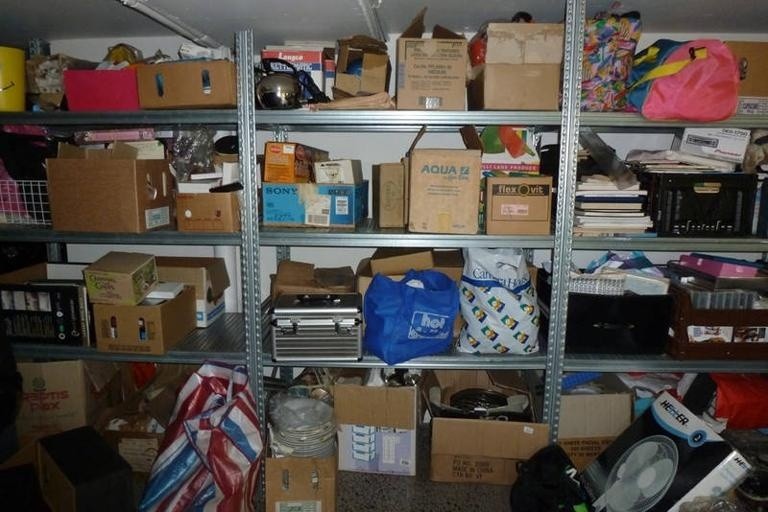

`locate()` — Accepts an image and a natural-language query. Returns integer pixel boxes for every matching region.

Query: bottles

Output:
[740,454,768,511]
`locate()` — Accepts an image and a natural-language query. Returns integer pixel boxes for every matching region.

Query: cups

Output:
[0,45,28,114]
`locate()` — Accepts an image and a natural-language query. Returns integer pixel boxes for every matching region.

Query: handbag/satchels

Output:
[454,246,542,357]
[360,263,462,367]
[134,358,268,512]
[624,32,743,124]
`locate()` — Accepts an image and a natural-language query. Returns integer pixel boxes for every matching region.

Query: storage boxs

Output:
[262,4,565,361]
[1,358,201,512]
[0,45,242,356]
[567,39,768,362]
[574,390,755,511]
[265,369,549,512]
[556,372,633,471]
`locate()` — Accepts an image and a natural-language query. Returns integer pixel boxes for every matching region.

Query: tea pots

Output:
[254,57,302,111]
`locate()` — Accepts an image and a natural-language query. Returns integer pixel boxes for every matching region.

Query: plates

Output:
[267,386,337,457]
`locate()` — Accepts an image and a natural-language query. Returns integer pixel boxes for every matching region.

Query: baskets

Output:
[0,179,55,226]
